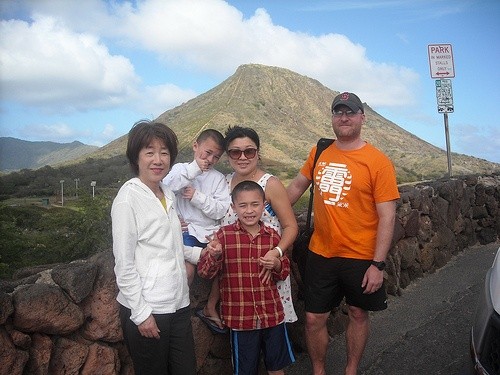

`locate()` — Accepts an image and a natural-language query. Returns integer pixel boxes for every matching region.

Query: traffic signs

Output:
[428,43,456,78]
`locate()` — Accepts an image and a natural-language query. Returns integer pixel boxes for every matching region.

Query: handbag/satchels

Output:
[292,228,316,262]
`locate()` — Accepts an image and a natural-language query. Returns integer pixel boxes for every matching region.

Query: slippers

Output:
[195,307,231,333]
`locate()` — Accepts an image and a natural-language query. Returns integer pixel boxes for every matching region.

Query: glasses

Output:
[226,147,258,159]
[334,110,362,117]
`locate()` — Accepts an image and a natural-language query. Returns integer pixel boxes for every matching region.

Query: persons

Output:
[161,129,232,333]
[285,92,400,375]
[225,126,299,323]
[110,120,197,375]
[197,180,296,375]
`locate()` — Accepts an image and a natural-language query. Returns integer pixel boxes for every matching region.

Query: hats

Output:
[332,92,364,113]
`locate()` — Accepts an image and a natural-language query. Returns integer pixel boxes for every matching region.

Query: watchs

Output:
[372,261,385,271]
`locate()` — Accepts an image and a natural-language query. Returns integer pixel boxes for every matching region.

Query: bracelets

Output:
[275,247,282,257]
